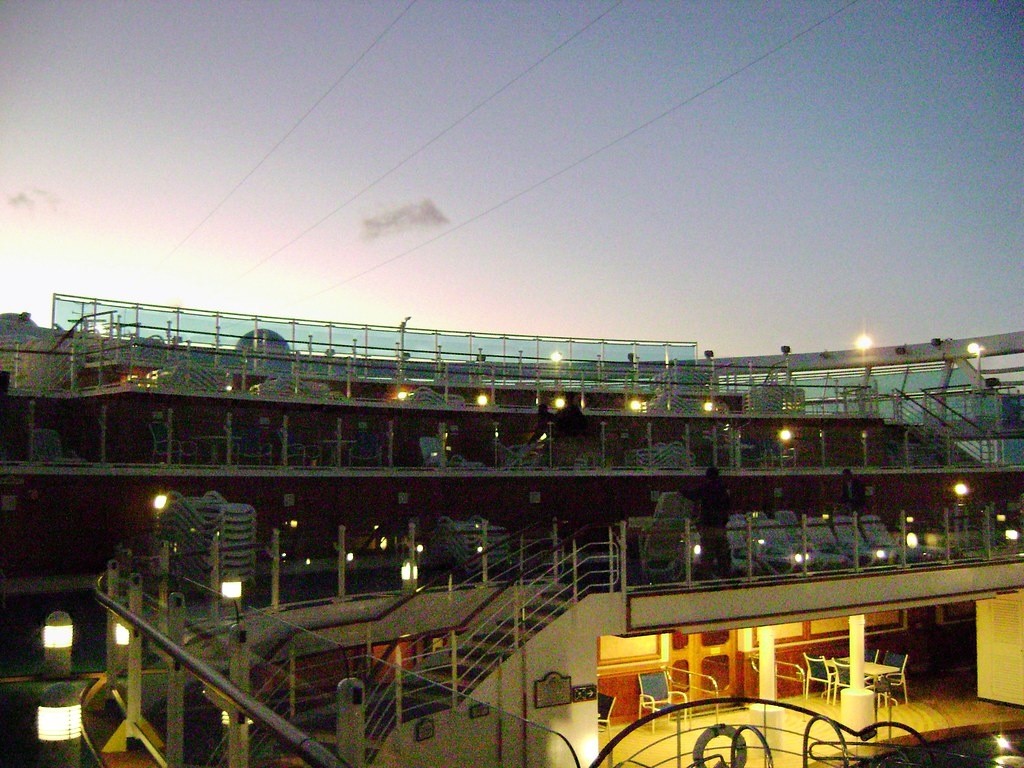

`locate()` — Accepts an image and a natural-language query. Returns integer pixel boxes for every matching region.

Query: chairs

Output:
[149,420,182,465]
[598,691,617,743]
[727,510,946,575]
[803,647,908,709]
[636,670,689,733]
[276,429,306,466]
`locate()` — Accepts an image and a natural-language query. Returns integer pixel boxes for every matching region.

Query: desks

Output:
[190,435,242,465]
[823,658,901,695]
[316,439,356,465]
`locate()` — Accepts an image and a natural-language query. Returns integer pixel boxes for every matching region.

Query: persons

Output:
[840,469,866,518]
[696,466,733,580]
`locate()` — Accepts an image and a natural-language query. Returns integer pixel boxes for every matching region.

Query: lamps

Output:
[221,573,242,618]
[35,684,81,768]
[114,619,131,667]
[400,558,419,591]
[41,611,74,679]
